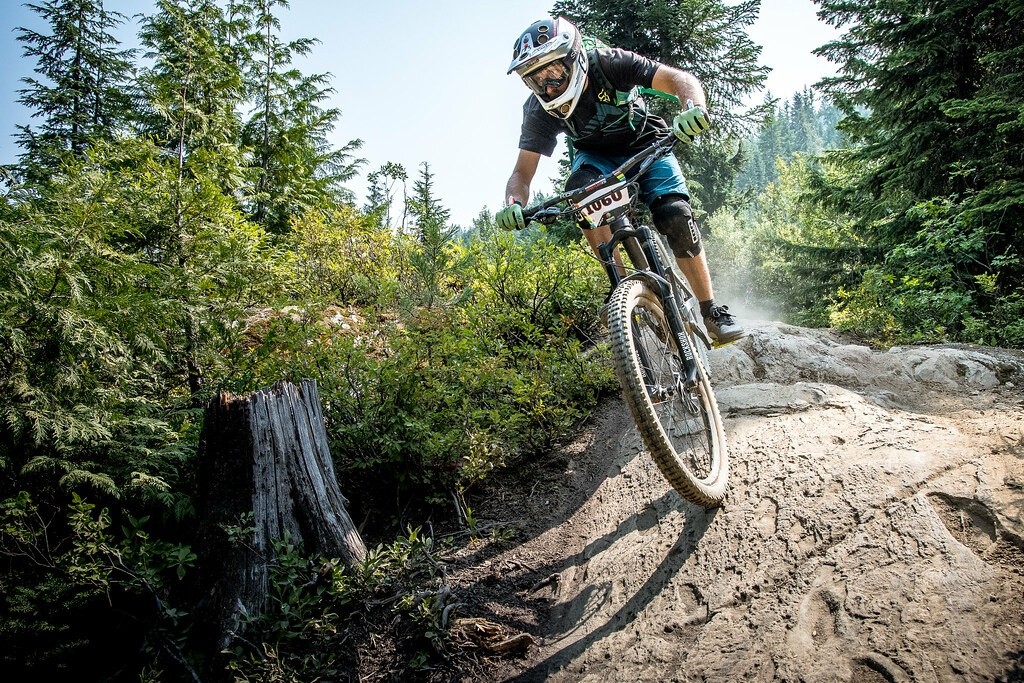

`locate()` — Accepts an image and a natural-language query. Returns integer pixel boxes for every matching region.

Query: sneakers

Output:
[703,305,746,345]
[599,303,609,329]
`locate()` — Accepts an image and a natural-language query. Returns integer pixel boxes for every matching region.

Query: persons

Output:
[495,17,746,345]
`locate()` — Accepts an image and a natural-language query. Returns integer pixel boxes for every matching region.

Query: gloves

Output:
[494,195,531,231]
[672,106,711,145]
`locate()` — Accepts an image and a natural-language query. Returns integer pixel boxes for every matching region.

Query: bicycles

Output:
[502,120,731,509]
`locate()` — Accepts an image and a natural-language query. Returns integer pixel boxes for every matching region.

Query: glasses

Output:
[522,28,581,95]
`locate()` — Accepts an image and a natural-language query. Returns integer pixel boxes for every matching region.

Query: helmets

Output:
[506,16,589,119]
